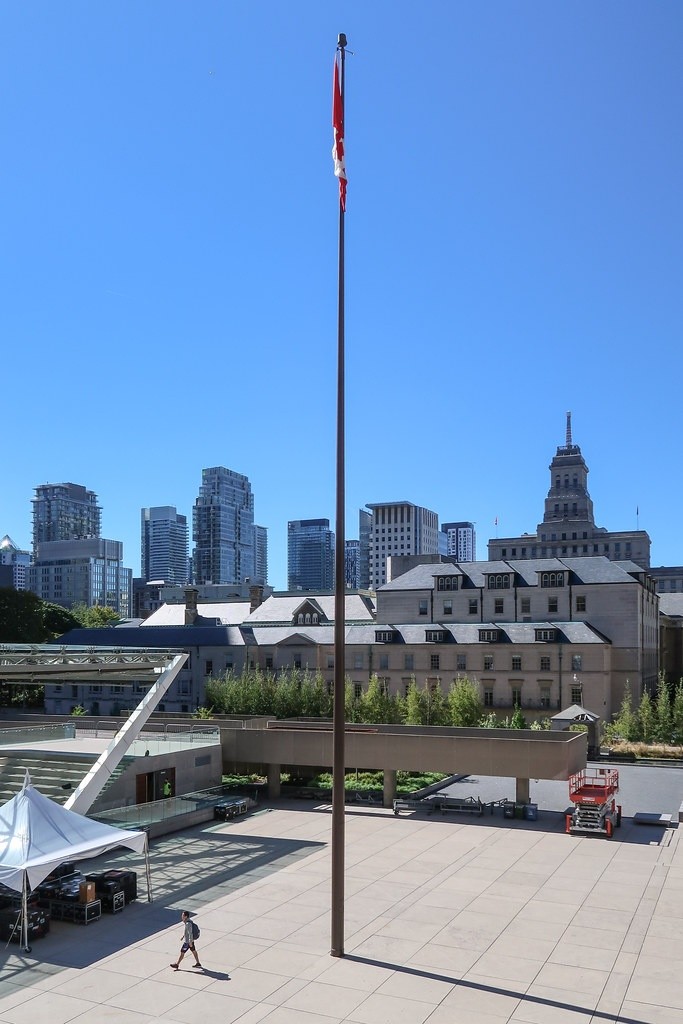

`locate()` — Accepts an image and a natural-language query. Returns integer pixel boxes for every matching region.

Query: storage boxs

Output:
[213,799,247,821]
[0,862,136,943]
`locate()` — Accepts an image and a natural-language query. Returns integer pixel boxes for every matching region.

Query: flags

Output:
[330,54,348,216]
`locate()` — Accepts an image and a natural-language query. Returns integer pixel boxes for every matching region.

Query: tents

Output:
[0,768,153,951]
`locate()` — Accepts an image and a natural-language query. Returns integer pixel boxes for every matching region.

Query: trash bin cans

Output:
[503,801,539,821]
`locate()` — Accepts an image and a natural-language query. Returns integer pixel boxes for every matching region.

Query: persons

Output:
[170,910,202,970]
[160,778,172,798]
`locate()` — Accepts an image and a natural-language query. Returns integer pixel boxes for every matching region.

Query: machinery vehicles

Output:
[565,767,622,838]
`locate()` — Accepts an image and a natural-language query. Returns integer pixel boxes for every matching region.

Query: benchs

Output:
[633,813,672,827]
[678,800,683,821]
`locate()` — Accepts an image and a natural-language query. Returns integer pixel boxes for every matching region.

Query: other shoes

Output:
[192,964,202,967]
[170,964,178,968]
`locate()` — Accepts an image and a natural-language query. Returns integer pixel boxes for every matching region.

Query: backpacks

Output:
[185,921,200,940]
